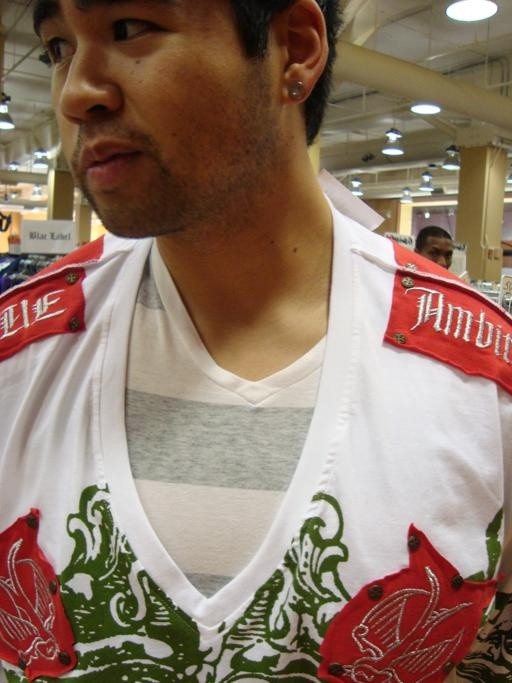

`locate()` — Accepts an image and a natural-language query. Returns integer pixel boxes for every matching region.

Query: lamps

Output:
[0,0,15,130]
[350,1,462,204]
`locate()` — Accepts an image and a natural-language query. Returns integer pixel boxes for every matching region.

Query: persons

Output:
[0,1,512,683]
[416,224,454,268]
